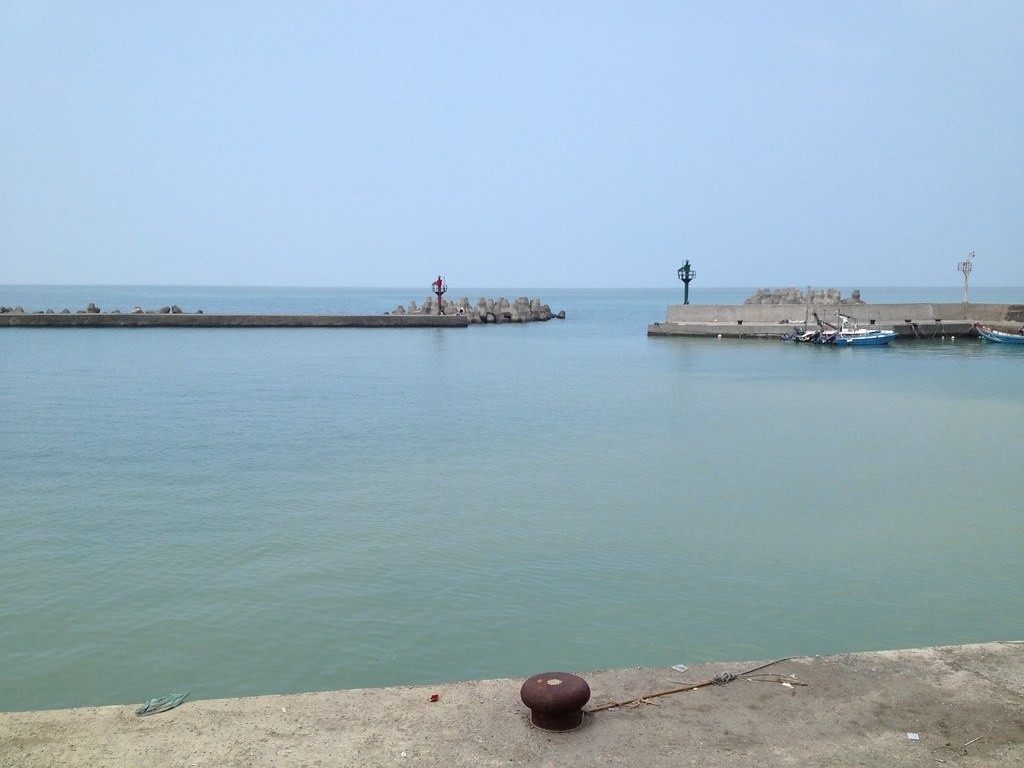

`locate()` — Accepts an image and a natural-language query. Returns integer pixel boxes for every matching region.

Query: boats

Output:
[780,329,804,340]
[796,329,869,343]
[975,322,1024,344]
[833,330,899,345]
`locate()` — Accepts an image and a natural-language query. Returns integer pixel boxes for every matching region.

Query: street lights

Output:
[432,275,448,316]
[678,260,696,305]
